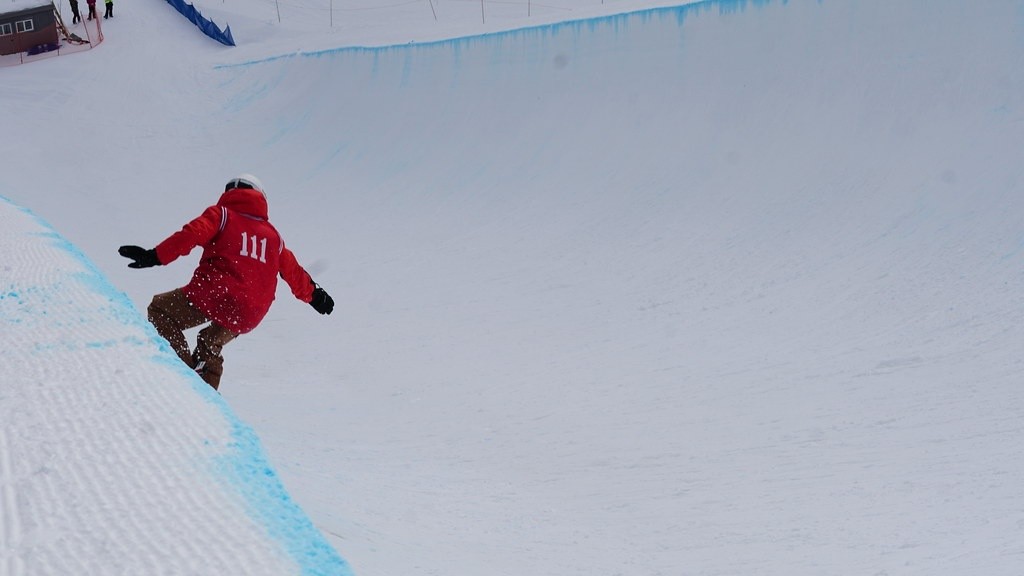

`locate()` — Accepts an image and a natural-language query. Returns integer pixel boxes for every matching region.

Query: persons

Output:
[86,0,97,21]
[103,0,113,19]
[69,0,82,24]
[119,175,335,390]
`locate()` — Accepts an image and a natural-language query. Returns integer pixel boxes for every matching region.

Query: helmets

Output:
[226,174,268,200]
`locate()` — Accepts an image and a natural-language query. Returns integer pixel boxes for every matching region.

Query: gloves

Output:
[118,245,161,269]
[309,283,335,315]
